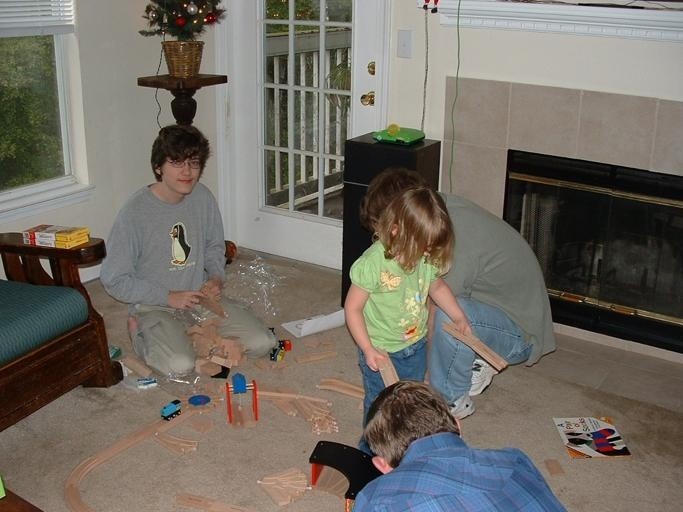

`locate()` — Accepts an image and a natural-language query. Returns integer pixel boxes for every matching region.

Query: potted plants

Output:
[135,0,224,78]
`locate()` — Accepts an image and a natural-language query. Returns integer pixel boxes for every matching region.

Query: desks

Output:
[136,74,227,125]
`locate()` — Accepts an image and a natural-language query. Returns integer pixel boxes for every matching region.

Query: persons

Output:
[350,380,566,511]
[359,168,557,422]
[98,123,276,377]
[343,185,472,456]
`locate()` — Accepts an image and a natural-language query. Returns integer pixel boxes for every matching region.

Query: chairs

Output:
[0,231,122,433]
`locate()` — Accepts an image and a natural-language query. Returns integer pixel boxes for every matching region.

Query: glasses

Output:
[164,158,202,169]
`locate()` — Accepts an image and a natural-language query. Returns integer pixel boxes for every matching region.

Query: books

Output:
[550,416,631,459]
[22,224,88,241]
[22,236,88,249]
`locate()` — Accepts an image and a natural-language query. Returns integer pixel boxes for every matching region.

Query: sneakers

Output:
[446,358,500,421]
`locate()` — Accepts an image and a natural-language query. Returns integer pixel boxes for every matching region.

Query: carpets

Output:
[0,246,645,511]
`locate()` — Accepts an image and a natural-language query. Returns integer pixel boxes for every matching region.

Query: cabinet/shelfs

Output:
[341,130,441,315]
